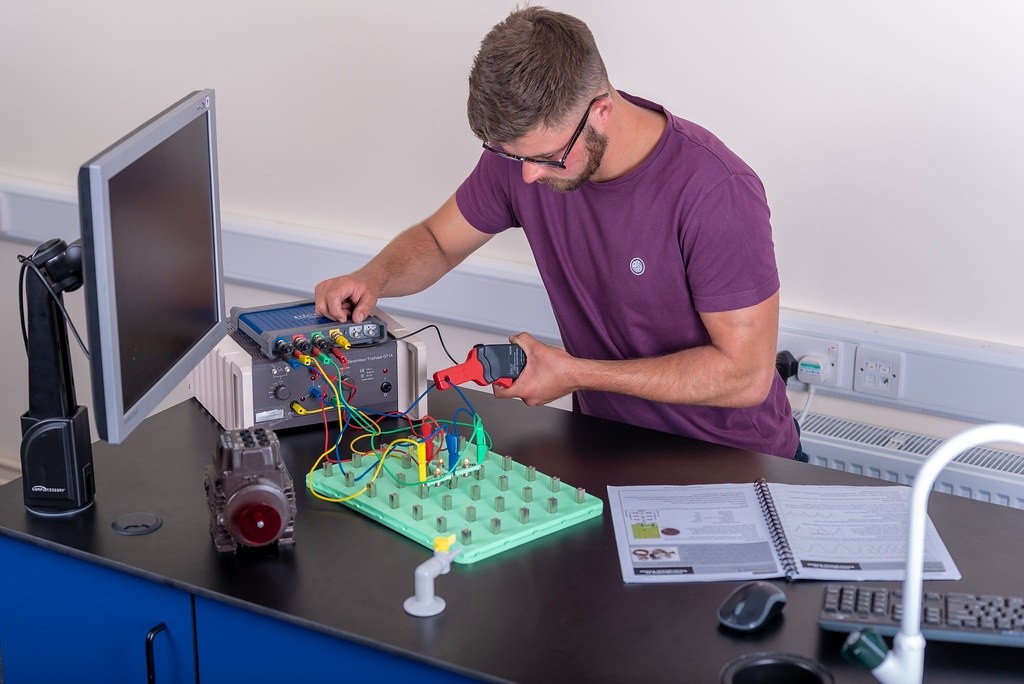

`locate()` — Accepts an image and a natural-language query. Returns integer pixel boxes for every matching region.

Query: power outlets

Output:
[775,332,843,389]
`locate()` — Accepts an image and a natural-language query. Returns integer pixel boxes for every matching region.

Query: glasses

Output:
[482,93,609,170]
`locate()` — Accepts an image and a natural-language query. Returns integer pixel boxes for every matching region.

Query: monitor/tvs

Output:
[18,88,228,521]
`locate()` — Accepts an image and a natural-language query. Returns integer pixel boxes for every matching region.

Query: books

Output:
[605,476,962,583]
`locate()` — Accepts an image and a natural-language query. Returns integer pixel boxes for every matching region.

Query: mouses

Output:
[717,579,788,636]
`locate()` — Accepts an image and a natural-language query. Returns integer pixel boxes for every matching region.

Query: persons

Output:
[315,7,803,462]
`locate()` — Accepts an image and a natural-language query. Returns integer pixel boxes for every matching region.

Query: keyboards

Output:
[816,583,1024,658]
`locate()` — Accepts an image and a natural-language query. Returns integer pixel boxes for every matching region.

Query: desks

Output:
[0,379,1024,684]
